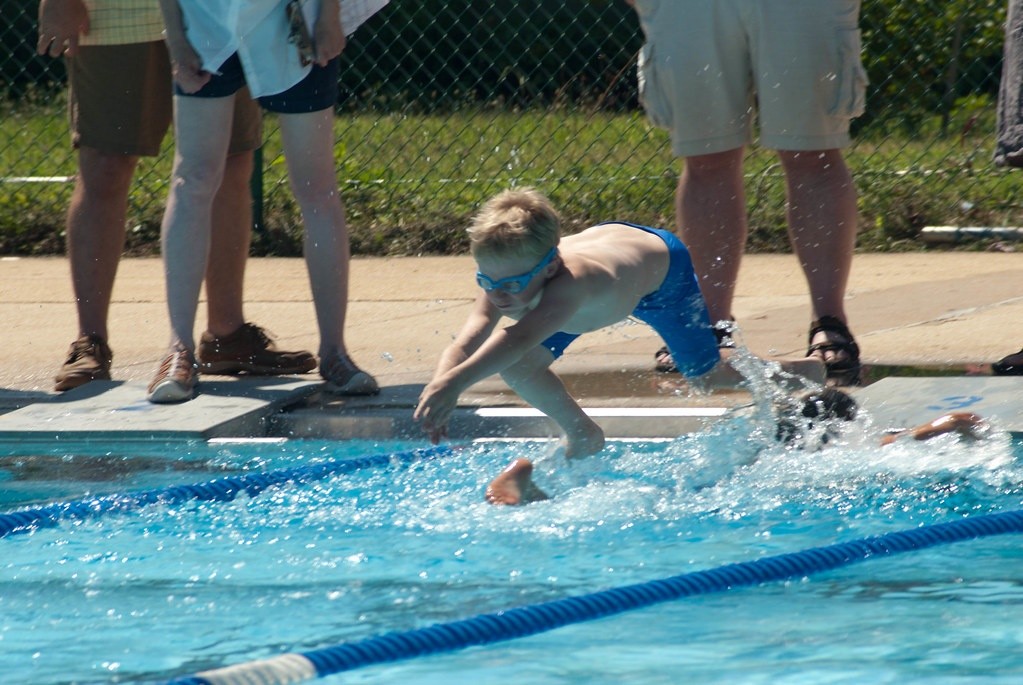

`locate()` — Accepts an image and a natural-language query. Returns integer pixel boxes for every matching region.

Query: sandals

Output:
[804,317,862,378]
[654,325,732,372]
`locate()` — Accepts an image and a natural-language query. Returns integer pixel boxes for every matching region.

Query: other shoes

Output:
[991,348,1023,375]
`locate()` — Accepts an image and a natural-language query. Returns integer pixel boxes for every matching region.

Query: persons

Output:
[37,0,381,404]
[412,188,827,460]
[485,386,980,505]
[631,0,868,379]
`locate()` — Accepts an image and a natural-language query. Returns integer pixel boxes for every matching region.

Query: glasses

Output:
[475,247,558,295]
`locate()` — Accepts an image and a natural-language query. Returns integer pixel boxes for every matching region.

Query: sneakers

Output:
[196,322,317,374]
[56,335,112,390]
[145,339,198,403]
[318,352,377,395]
